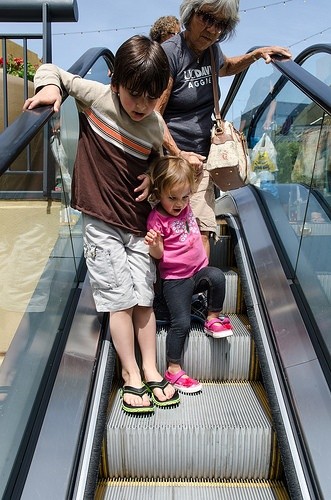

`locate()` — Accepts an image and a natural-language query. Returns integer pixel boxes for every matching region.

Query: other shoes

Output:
[165,369,203,394]
[203,317,234,338]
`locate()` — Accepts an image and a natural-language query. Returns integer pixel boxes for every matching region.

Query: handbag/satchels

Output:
[291,126,331,185]
[207,119,251,191]
[251,133,279,172]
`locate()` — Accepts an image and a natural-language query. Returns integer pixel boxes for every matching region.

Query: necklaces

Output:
[191,46,203,64]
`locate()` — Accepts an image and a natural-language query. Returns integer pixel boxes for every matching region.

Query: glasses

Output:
[194,7,228,32]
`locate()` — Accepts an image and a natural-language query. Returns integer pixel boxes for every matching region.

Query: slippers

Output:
[143,377,181,405]
[120,385,155,412]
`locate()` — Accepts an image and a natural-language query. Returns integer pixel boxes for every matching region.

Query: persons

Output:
[145,155,234,394]
[149,14,180,46]
[152,0,293,324]
[22,35,206,414]
